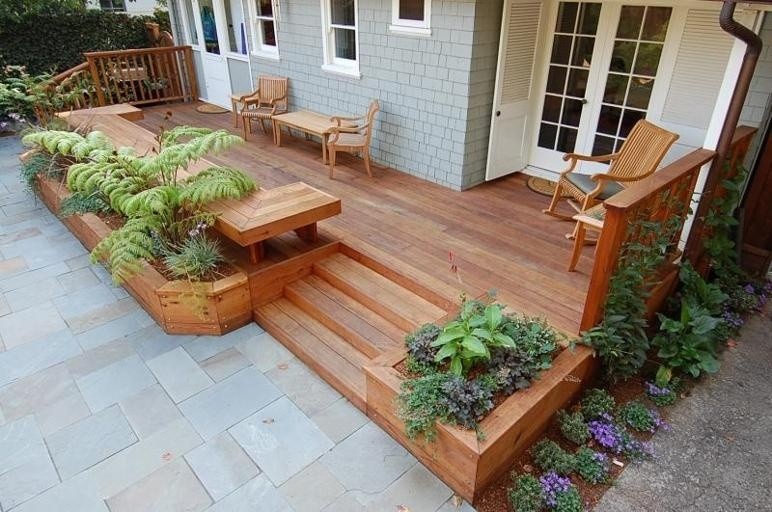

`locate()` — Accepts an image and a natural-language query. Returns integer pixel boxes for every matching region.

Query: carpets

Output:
[195,103,229,113]
[528,177,576,198]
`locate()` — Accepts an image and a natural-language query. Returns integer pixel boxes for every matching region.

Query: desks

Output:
[567,203,607,273]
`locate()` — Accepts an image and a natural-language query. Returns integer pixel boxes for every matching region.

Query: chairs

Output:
[541,116,680,245]
[229,74,380,180]
